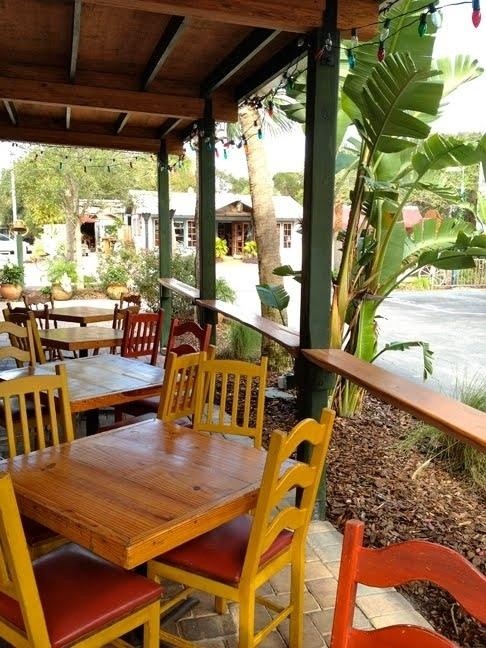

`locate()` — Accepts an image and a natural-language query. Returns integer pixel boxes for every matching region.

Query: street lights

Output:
[140,213,152,280]
[169,208,177,257]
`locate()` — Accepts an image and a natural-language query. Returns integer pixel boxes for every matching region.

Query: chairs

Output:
[0,361,75,464]
[191,351,269,454]
[0,290,216,438]
[329,516,485,647]
[1,469,166,648]
[146,407,338,648]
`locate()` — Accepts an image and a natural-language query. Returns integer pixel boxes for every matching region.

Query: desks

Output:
[0,417,306,631]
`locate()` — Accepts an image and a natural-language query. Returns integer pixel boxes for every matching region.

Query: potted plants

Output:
[0,253,129,300]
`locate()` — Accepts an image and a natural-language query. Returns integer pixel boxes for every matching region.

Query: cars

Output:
[0,234,32,254]
